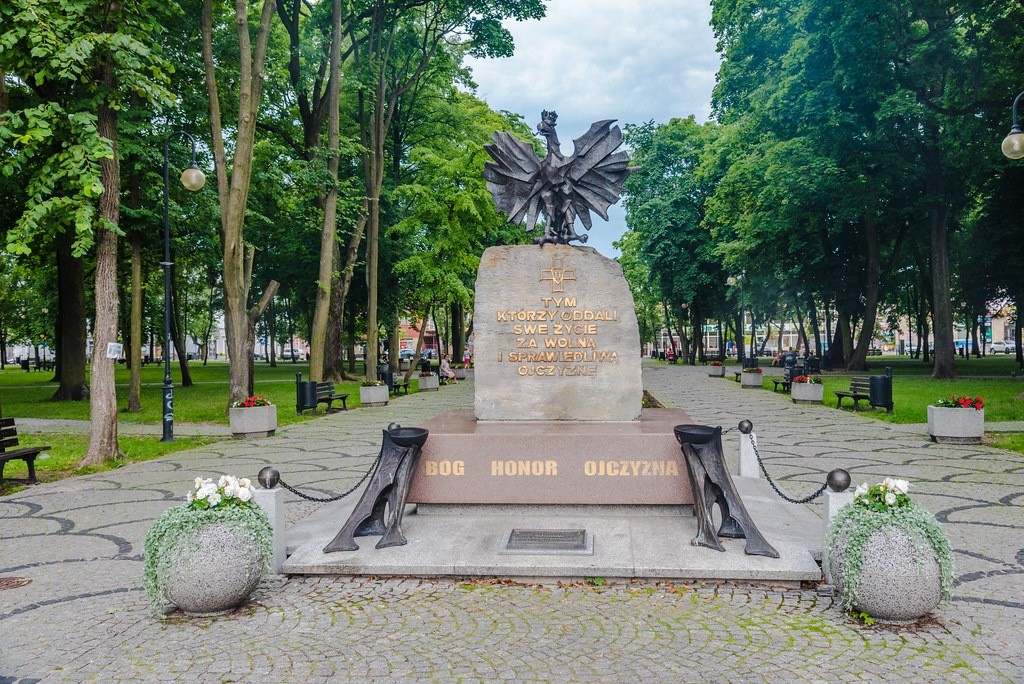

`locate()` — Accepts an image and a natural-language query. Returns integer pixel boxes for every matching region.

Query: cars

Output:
[754,341,773,357]
[989,339,1024,355]
[400,348,437,359]
[254,348,305,361]
[905,341,935,355]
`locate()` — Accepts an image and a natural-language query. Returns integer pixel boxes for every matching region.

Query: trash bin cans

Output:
[422,361,430,372]
[660,352,665,357]
[378,372,393,390]
[789,366,802,389]
[746,358,759,369]
[869,367,894,406]
[296,372,317,407]
[652,351,655,356]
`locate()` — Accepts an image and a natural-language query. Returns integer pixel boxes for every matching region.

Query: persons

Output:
[379,355,389,364]
[807,352,815,360]
[785,347,796,355]
[667,348,674,355]
[440,354,459,385]
[772,350,784,366]
[306,352,310,361]
[464,347,472,370]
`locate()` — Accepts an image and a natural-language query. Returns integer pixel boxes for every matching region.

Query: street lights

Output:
[160,130,206,443]
[681,302,693,365]
[727,270,747,369]
[41,307,49,370]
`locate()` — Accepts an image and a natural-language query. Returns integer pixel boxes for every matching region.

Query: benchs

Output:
[393,372,409,395]
[440,375,449,385]
[317,382,350,413]
[834,376,870,410]
[734,372,741,381]
[772,367,791,392]
[0,418,51,485]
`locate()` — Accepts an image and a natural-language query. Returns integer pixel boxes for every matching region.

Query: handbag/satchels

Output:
[462,357,465,361]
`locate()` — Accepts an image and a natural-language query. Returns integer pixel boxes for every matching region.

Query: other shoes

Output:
[453,381,459,384]
[444,381,448,385]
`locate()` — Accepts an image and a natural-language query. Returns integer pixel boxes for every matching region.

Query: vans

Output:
[953,339,973,354]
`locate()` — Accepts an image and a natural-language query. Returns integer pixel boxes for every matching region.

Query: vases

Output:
[741,372,762,385]
[418,375,439,389]
[709,366,725,375]
[791,382,823,400]
[360,384,389,403]
[229,405,277,433]
[453,369,466,378]
[927,405,984,437]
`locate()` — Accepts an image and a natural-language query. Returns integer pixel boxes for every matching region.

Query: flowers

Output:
[710,361,722,366]
[793,374,822,384]
[742,368,763,374]
[934,394,984,411]
[361,379,384,386]
[233,396,271,407]
[455,365,465,369]
[140,474,275,618]
[419,371,436,377]
[824,477,955,615]
